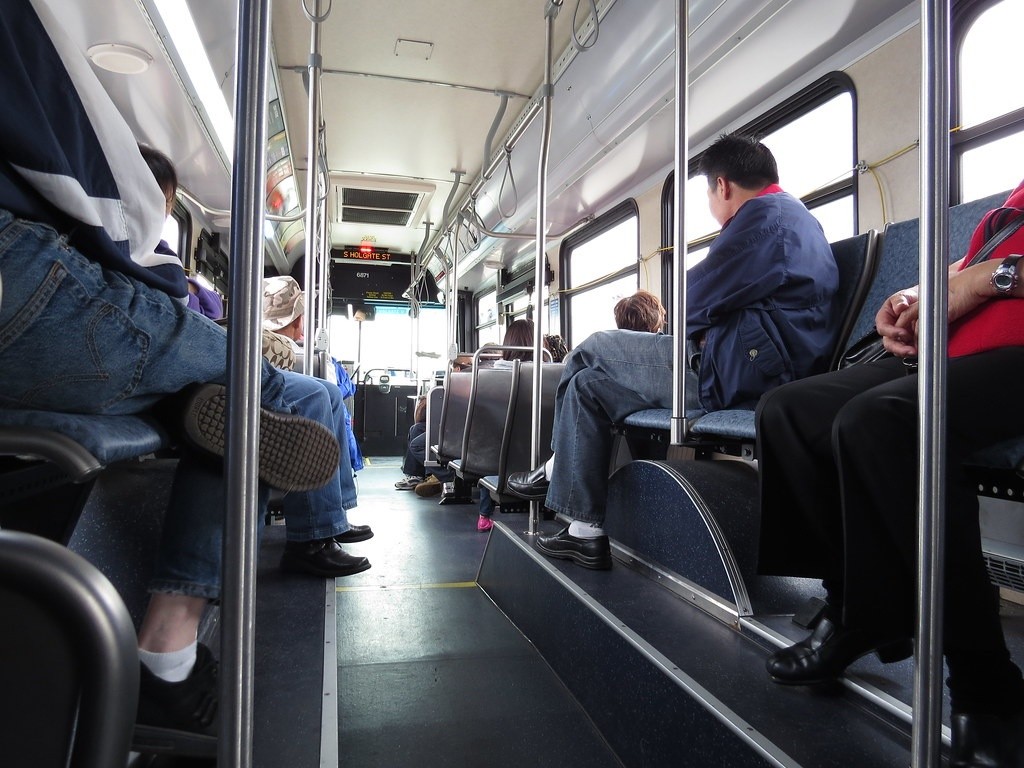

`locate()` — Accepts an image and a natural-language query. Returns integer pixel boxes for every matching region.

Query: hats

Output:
[262,275,317,330]
[454,356,473,366]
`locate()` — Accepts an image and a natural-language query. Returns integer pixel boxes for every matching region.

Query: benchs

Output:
[625,185,1024,477]
[423,344,568,506]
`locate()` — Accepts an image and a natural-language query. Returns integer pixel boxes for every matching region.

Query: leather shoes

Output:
[280,536,372,578]
[507,461,551,499]
[949,662,1024,768]
[325,523,373,543]
[536,522,613,571]
[765,617,915,687]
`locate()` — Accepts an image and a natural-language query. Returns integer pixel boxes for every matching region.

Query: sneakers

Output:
[394,475,423,490]
[441,481,455,497]
[415,473,442,497]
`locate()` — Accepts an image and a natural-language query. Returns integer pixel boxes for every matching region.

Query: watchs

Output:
[989,253,1023,298]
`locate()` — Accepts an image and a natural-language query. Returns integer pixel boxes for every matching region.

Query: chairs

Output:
[0,403,164,481]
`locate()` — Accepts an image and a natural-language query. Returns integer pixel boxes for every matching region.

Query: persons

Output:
[352,306,374,321]
[754,180,1024,768]
[615,292,670,338]
[506,135,841,571]
[476,319,568,531]
[0,0,374,768]
[394,340,504,498]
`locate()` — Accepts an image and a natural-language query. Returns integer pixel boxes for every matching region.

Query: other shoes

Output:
[478,514,493,531]
[130,639,223,750]
[185,383,341,491]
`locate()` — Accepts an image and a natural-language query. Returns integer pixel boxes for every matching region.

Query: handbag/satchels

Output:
[212,316,296,371]
[838,325,893,372]
